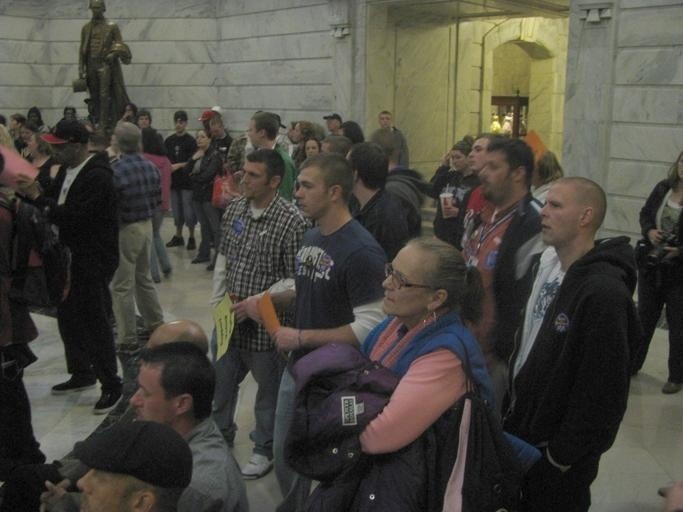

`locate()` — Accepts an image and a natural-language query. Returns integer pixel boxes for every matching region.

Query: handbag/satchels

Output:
[212,176,235,211]
[431,331,520,512]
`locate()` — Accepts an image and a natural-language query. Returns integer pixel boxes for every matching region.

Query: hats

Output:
[271,114,287,128]
[198,111,214,121]
[39,119,89,144]
[77,421,194,486]
[323,113,341,119]
[174,111,188,121]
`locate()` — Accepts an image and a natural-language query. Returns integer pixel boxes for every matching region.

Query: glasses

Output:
[383,261,430,290]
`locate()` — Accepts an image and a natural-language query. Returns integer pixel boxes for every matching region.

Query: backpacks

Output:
[2,197,71,306]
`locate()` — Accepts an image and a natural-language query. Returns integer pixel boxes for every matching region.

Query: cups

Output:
[438,192,453,220]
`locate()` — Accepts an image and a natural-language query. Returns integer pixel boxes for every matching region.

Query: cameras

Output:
[643,231,680,270]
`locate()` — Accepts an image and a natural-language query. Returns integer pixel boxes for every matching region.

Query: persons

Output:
[2,105,683,512]
[78,2,137,130]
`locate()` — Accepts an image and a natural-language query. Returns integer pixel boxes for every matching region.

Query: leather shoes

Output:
[166,236,213,270]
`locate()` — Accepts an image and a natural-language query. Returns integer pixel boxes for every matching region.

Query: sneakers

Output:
[52,375,97,396]
[243,452,274,481]
[93,380,123,414]
